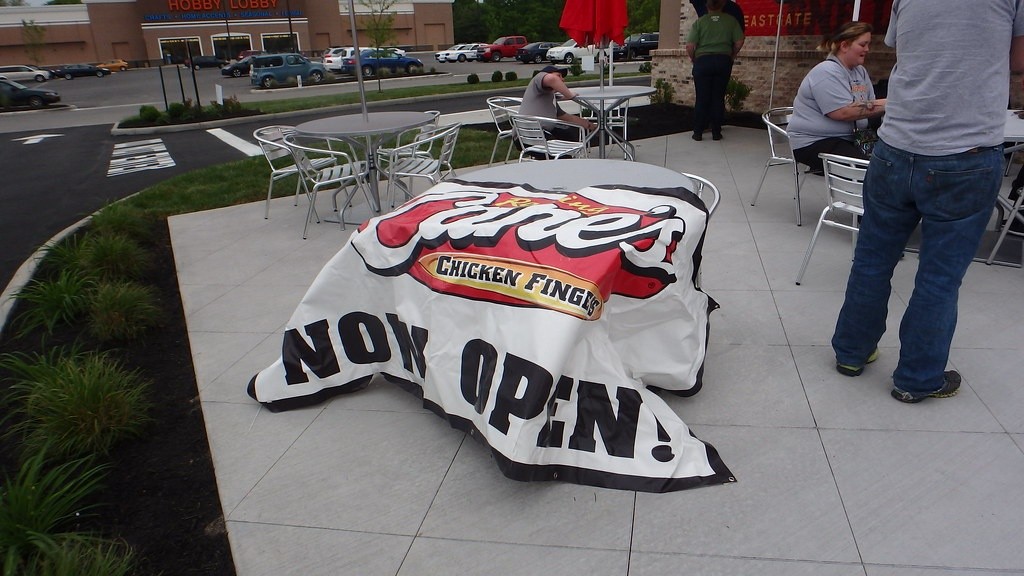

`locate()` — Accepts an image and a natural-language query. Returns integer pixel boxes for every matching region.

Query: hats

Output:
[539,66,568,78]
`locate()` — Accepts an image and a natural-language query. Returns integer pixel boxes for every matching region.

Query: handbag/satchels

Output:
[853,129,879,169]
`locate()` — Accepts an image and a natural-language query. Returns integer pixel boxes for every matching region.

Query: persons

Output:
[512,66,596,159]
[831,0,1024,402]
[686,0,746,140]
[787,21,887,180]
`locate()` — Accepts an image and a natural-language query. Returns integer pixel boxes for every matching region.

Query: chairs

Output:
[750,106,1024,285]
[253,96,720,291]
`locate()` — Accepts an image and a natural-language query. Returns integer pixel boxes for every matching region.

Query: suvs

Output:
[236,50,269,61]
[613,32,660,62]
[545,39,611,64]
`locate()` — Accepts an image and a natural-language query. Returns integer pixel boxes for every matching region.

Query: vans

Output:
[0,65,50,83]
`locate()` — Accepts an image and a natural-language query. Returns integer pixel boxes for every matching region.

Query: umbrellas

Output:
[559,0,629,159]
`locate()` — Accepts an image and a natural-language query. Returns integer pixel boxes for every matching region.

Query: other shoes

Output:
[713,132,723,140]
[692,128,703,141]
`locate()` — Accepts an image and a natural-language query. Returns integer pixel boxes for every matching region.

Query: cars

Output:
[222,55,273,78]
[57,64,112,80]
[340,49,423,77]
[444,43,487,62]
[28,65,56,79]
[320,47,406,72]
[96,58,129,72]
[517,42,563,64]
[184,56,231,70]
[0,76,61,110]
[249,53,327,89]
[434,45,465,63]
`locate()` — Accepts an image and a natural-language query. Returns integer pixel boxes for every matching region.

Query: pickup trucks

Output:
[476,35,528,62]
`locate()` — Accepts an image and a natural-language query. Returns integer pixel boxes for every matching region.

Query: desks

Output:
[881,108,1024,230]
[553,85,657,162]
[438,159,701,245]
[297,112,435,225]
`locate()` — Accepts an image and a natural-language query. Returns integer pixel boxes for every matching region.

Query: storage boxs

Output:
[635,103,669,127]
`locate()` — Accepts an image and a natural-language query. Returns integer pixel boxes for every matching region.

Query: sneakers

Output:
[891,369,962,403]
[836,345,877,377]
